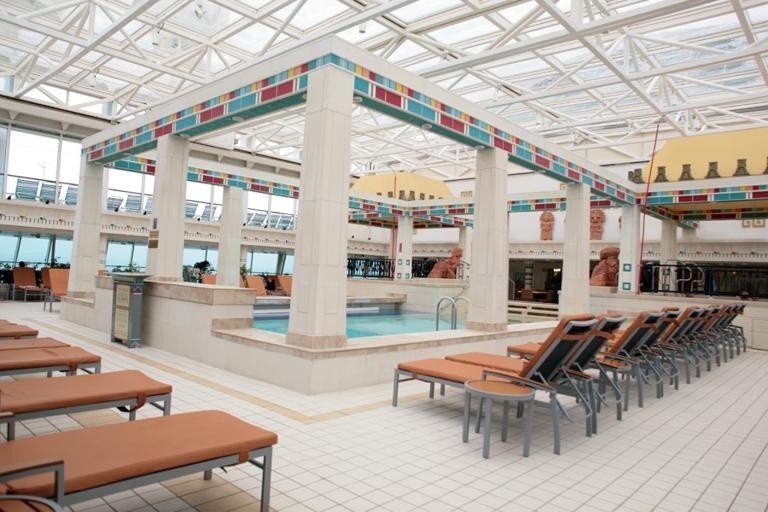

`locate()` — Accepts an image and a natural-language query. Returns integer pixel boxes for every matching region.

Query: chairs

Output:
[600,308,680,422]
[0,369,172,441]
[516,285,555,302]
[0,409,277,511]
[396,313,626,459]
[662,302,747,384]
[12,266,49,301]
[44,268,70,312]
[39,267,51,300]
[1,320,102,379]
[201,271,292,297]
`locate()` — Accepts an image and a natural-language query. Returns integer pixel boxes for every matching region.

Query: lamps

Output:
[192,0,209,19]
